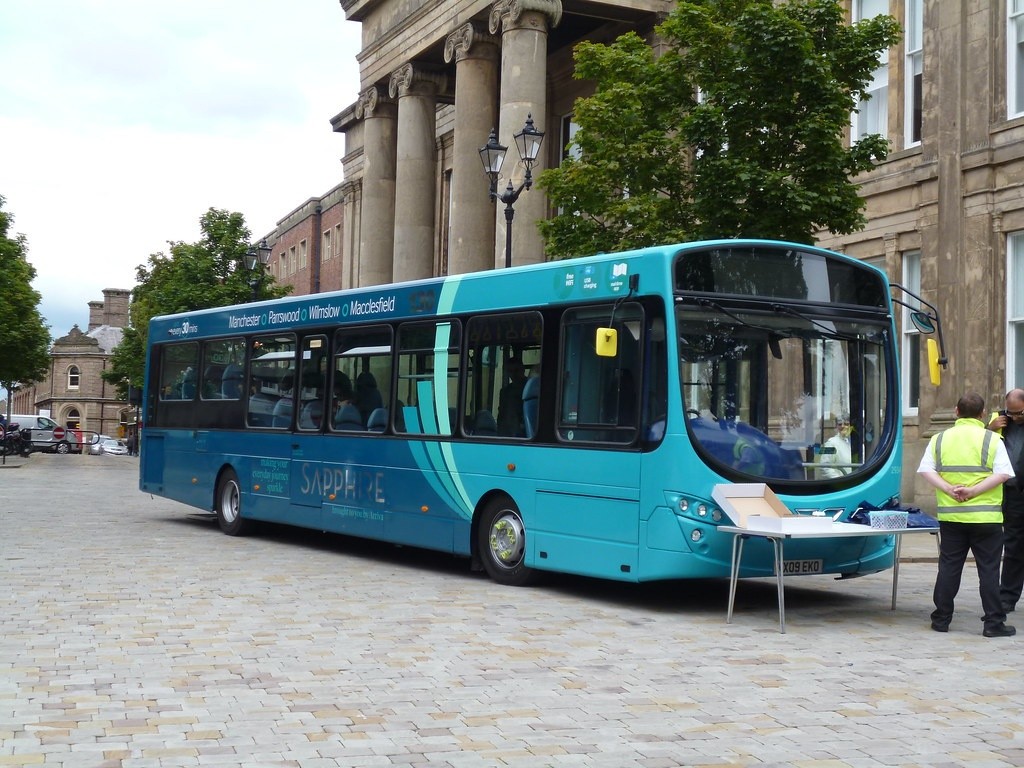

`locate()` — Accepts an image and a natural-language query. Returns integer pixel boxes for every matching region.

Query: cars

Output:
[100,439,130,455]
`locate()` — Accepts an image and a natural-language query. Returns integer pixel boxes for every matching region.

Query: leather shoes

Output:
[931,622,948,632]
[983,624,1015,637]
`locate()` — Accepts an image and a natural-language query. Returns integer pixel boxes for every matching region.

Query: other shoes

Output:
[999,602,1015,611]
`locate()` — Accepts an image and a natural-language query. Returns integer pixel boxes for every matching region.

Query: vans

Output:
[1,414,80,455]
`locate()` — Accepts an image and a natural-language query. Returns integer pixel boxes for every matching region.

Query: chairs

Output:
[165,364,540,439]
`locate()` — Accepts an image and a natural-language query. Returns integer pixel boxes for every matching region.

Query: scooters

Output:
[0,413,35,457]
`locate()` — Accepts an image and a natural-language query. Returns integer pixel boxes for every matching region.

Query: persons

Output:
[917,392,1016,638]
[331,395,352,429]
[981,389,1024,621]
[820,414,865,479]
[127,435,133,455]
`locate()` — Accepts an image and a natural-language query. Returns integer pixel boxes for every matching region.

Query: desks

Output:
[716,523,940,635]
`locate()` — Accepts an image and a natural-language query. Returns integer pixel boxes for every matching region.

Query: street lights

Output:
[477,110,547,268]
[241,238,273,303]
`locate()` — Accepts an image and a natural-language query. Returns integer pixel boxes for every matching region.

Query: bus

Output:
[140,239,950,588]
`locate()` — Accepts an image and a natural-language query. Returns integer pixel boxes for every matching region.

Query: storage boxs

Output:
[712,482,834,532]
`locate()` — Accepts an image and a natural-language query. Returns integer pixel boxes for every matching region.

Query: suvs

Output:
[89,435,112,455]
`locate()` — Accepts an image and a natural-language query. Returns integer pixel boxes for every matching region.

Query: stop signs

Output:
[53,427,65,439]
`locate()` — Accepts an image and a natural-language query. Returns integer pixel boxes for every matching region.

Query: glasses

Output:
[837,423,849,427]
[1005,408,1024,417]
